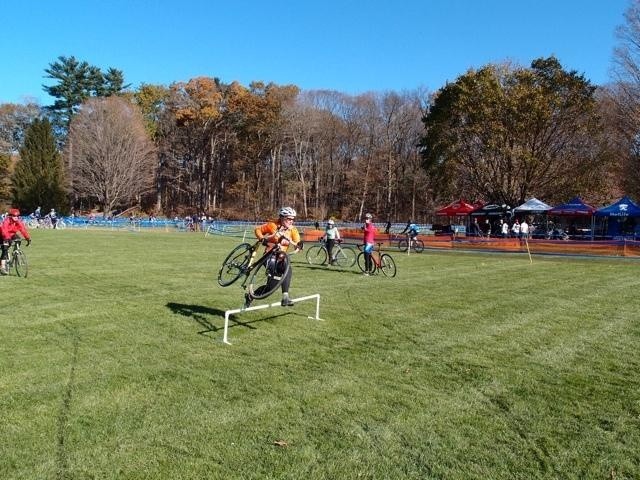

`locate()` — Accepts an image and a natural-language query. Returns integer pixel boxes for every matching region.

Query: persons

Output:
[384,219,391,232]
[362,212,375,276]
[315,221,319,230]
[401,219,419,247]
[321,220,342,266]
[254,206,304,306]
[0,209,30,275]
[30,206,213,233]
[472,216,569,240]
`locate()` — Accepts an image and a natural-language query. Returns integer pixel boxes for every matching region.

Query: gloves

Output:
[298,241,304,249]
[261,238,267,246]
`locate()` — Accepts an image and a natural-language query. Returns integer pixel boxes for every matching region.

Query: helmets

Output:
[8,209,20,216]
[328,219,334,225]
[365,213,372,218]
[278,207,297,217]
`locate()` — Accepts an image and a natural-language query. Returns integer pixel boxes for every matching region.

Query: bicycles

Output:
[396,232,424,254]
[26,216,67,230]
[218,229,303,302]
[1,236,32,278]
[306,234,357,271]
[355,243,397,279]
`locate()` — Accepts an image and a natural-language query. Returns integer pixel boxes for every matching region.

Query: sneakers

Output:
[245,293,252,307]
[281,299,294,305]
[0,269,8,275]
